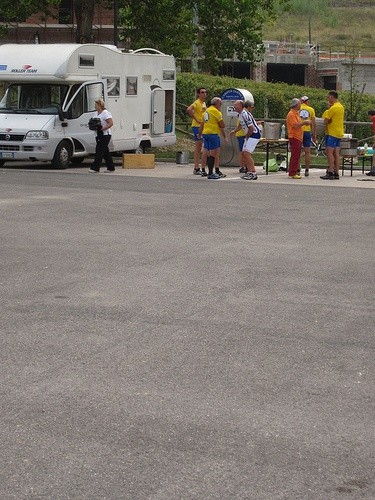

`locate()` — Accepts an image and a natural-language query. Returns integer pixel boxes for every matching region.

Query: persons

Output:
[229,100,262,180]
[319,91,344,180]
[186,88,208,175]
[88,99,116,173]
[287,98,312,179]
[199,97,228,179]
[236,100,264,173]
[365,109,375,177]
[297,96,316,177]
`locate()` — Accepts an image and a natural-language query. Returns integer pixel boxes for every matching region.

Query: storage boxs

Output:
[122,153,155,169]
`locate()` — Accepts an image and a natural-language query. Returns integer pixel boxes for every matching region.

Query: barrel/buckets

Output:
[175,151,189,164]
[263,123,281,140]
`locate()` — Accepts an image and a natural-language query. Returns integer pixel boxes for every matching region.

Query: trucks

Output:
[1,42,177,170]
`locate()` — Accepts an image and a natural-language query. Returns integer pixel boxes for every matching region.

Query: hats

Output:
[302,96,309,101]
[289,98,300,107]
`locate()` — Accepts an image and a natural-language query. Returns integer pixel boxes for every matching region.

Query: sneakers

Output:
[320,172,339,180]
[104,169,115,172]
[239,167,258,180]
[288,170,309,179]
[193,169,226,179]
[88,170,98,173]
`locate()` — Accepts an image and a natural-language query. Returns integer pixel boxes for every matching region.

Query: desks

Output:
[258,137,290,175]
[341,154,374,176]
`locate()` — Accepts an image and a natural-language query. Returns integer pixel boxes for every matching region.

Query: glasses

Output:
[251,107,254,108]
[201,91,206,93]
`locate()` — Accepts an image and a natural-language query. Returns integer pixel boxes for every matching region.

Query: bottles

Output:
[281,124,286,138]
[256,122,262,138]
[364,143,369,155]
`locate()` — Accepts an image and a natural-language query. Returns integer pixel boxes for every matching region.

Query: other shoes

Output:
[365,169,375,176]
